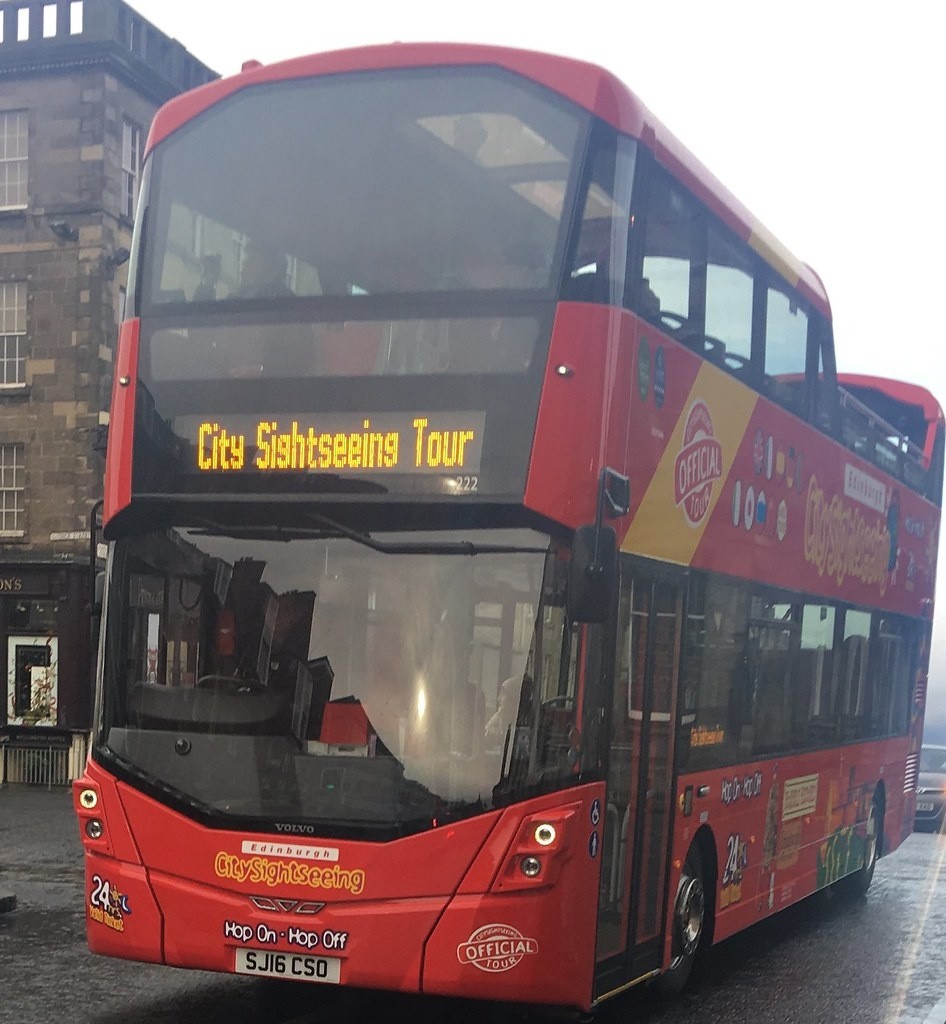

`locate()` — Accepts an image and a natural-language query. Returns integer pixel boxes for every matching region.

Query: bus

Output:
[71,39,946,1015]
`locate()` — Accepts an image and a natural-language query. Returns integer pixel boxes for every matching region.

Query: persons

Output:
[481,672,543,762]
[182,240,308,379]
[226,586,306,698]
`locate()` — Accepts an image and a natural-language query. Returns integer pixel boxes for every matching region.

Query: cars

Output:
[909,743,946,835]
